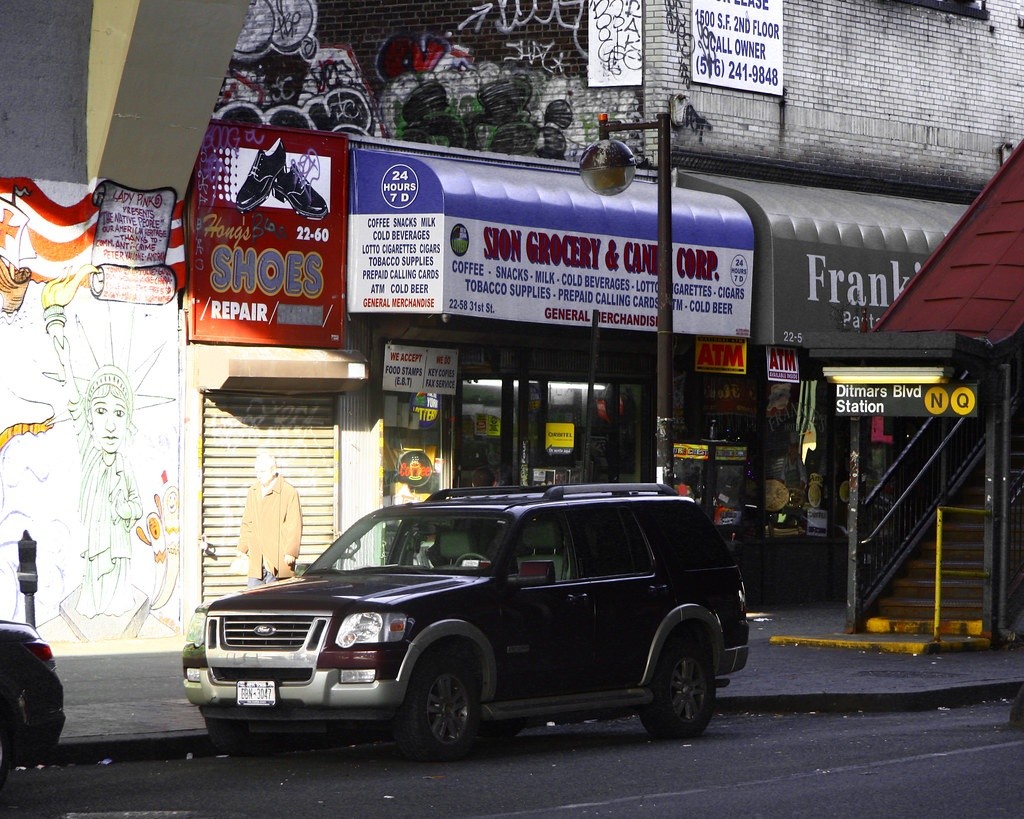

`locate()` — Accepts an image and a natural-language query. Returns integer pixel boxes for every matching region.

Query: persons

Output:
[237,453,302,588]
[471,465,498,488]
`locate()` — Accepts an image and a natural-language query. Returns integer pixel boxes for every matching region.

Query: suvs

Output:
[182,482,750,762]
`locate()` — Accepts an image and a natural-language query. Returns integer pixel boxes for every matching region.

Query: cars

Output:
[0,619,66,790]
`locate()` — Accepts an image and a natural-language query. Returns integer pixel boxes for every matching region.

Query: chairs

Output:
[438,530,475,565]
[516,521,564,578]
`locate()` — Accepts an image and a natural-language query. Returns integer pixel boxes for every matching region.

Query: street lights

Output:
[578,113,676,490]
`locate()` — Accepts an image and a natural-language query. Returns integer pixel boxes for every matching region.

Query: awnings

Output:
[676,168,973,350]
[346,146,755,340]
